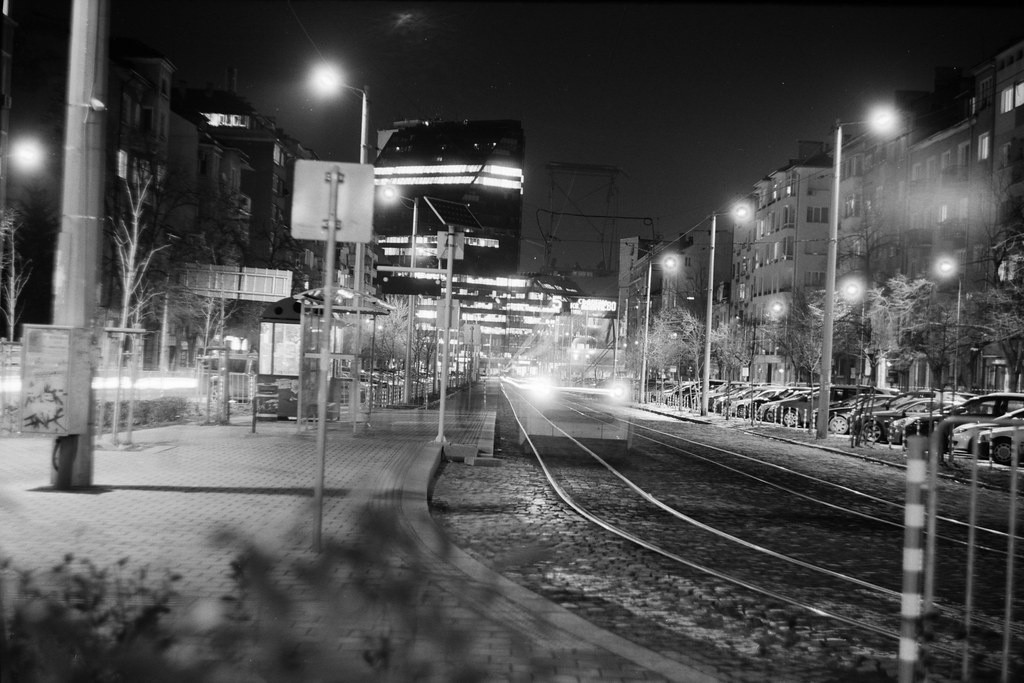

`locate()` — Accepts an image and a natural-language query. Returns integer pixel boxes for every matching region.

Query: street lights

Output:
[641,258,676,404]
[317,67,369,435]
[943,263,962,393]
[701,207,747,417]
[386,187,419,404]
[849,284,864,386]
[818,115,893,438]
[775,304,788,385]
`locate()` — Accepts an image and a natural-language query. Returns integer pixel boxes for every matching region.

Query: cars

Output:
[646,378,810,422]
[948,408,1024,466]
[847,388,985,445]
[338,371,464,400]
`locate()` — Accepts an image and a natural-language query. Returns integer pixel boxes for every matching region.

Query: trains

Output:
[502,293,640,463]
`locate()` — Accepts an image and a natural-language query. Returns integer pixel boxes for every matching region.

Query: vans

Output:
[902,392,1024,454]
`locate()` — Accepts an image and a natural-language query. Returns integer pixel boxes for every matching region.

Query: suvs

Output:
[767,383,891,428]
